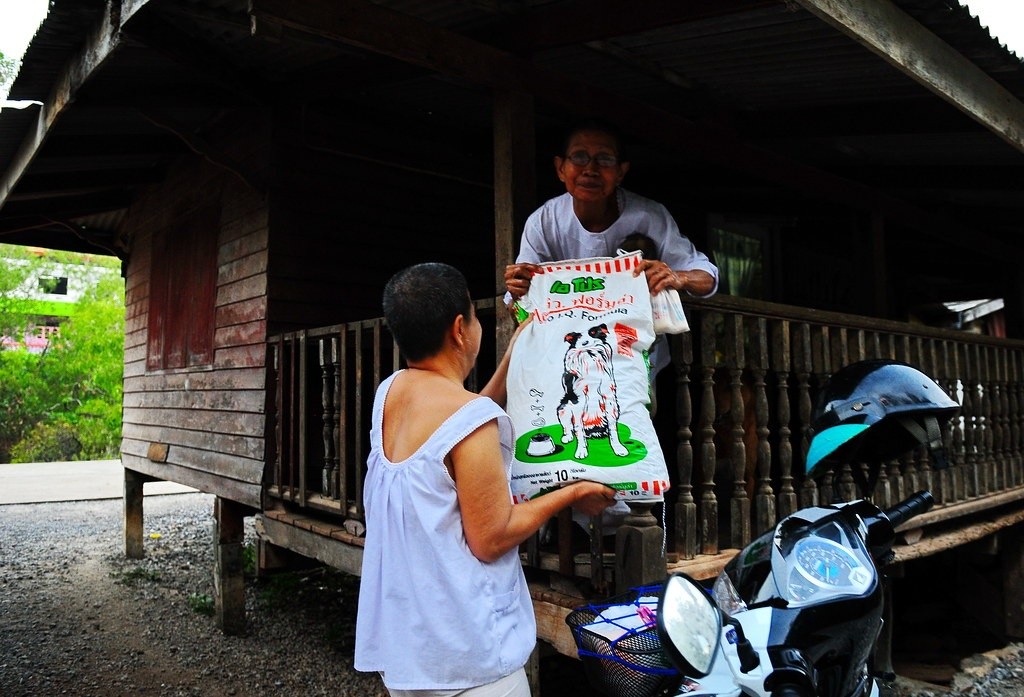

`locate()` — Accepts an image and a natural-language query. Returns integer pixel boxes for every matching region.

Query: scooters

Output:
[564,489,934,697]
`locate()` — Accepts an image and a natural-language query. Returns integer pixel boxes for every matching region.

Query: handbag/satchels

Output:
[616,248,690,335]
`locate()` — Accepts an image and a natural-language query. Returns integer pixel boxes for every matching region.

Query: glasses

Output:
[564,151,623,169]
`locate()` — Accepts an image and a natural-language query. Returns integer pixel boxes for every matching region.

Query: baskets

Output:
[566,579,715,697]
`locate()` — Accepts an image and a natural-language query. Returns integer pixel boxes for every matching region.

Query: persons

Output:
[359,262,618,697]
[502,107,719,536]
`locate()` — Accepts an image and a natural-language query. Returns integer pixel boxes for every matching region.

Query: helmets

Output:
[804,358,961,473]
